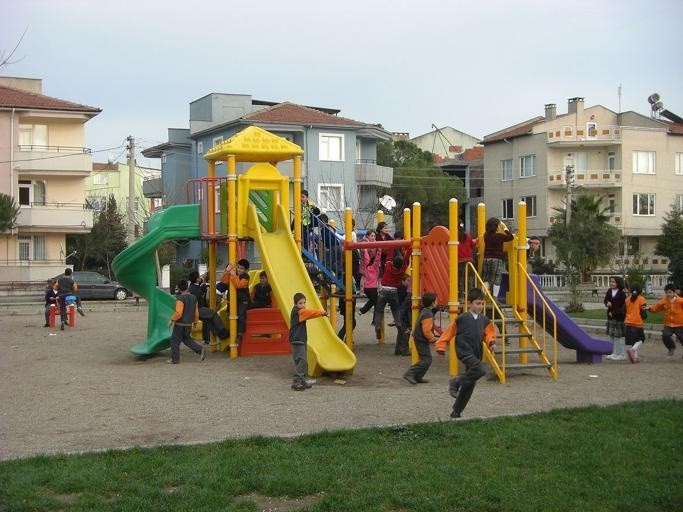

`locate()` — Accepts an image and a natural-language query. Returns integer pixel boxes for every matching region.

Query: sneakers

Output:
[666,346,676,356]
[199,347,206,362]
[41,324,49,328]
[447,376,458,398]
[290,379,313,391]
[483,293,501,306]
[63,321,68,325]
[60,324,64,330]
[399,372,430,385]
[604,348,642,364]
[448,411,461,418]
[357,308,411,357]
[166,360,174,364]
[217,327,230,341]
[75,307,85,316]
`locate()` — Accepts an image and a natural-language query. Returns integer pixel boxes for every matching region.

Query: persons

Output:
[290,190,413,356]
[53,268,84,331]
[44,279,69,328]
[166,259,271,365]
[603,276,683,364]
[288,293,330,392]
[402,290,437,386]
[434,289,497,419]
[456,217,541,305]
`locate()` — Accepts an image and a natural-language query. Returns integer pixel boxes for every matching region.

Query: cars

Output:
[44,268,133,302]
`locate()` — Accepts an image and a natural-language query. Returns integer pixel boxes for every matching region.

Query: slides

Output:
[111,227,178,355]
[497,271,612,361]
[248,204,356,377]
